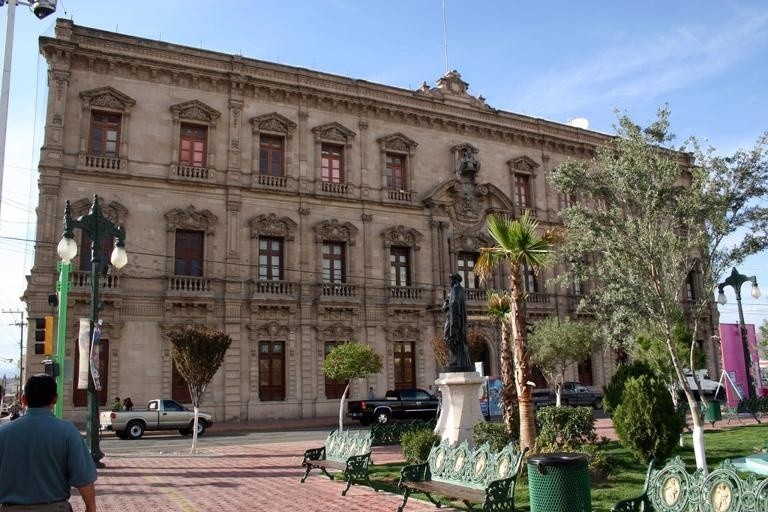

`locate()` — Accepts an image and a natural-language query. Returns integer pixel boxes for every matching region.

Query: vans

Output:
[676,368,726,400]
[0,394,16,415]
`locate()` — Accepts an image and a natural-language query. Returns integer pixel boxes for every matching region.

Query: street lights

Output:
[58,194,128,468]
[718,266,761,396]
[1,2,59,213]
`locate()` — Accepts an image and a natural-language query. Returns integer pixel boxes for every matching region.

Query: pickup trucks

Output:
[346,388,442,425]
[99,398,213,440]
[531,382,604,411]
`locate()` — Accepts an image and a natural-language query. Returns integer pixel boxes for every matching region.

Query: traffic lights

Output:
[34,315,58,379]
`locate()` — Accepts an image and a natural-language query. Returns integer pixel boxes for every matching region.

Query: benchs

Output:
[611,454,767,512]
[727,407,763,424]
[301,429,382,498]
[397,437,530,511]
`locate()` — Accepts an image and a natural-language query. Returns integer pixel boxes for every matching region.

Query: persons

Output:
[441,272,473,369]
[368,387,376,400]
[0,373,98,511]
[120,399,127,411]
[111,397,121,410]
[125,397,133,411]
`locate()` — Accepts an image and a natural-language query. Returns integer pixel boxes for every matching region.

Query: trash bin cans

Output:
[525,451,591,511]
[703,399,723,427]
